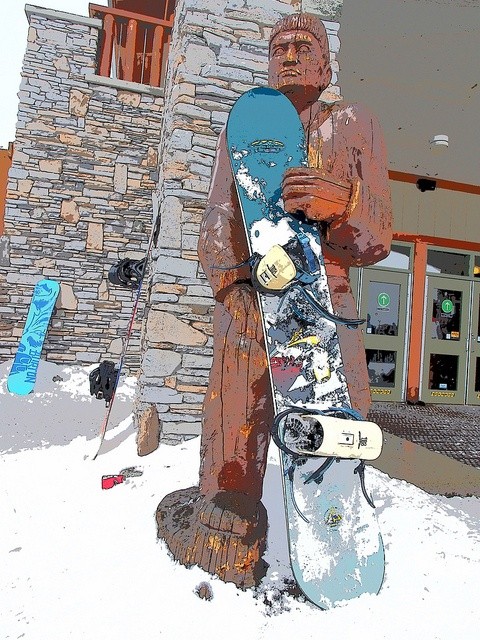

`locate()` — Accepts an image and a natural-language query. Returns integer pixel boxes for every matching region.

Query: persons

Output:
[197,13,393,584]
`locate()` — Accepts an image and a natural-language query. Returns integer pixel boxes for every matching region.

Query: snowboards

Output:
[6,279,61,396]
[227,86,385,611]
[89,199,162,460]
